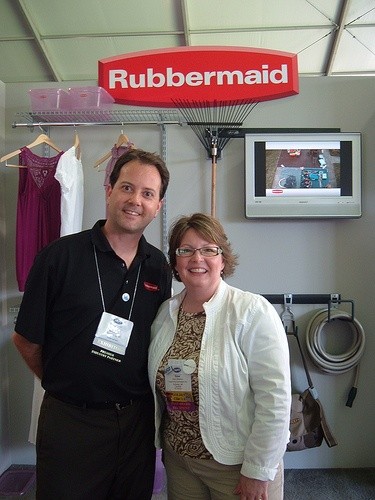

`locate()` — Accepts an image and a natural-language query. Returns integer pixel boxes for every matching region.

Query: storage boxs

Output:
[28,89,70,121]
[0,469,36,500]
[67,86,115,121]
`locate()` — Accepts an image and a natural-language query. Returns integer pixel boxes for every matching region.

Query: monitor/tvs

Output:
[244,133,361,217]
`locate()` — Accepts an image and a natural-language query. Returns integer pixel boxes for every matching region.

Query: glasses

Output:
[176,245,223,257]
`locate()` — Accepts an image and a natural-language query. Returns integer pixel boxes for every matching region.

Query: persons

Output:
[147,214,291,500]
[12,150,171,500]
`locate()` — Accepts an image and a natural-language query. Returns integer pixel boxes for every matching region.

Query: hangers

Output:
[93,122,138,173]
[0,122,62,168]
[73,121,80,160]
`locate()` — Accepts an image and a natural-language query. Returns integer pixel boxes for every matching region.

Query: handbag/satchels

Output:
[283,331,338,452]
